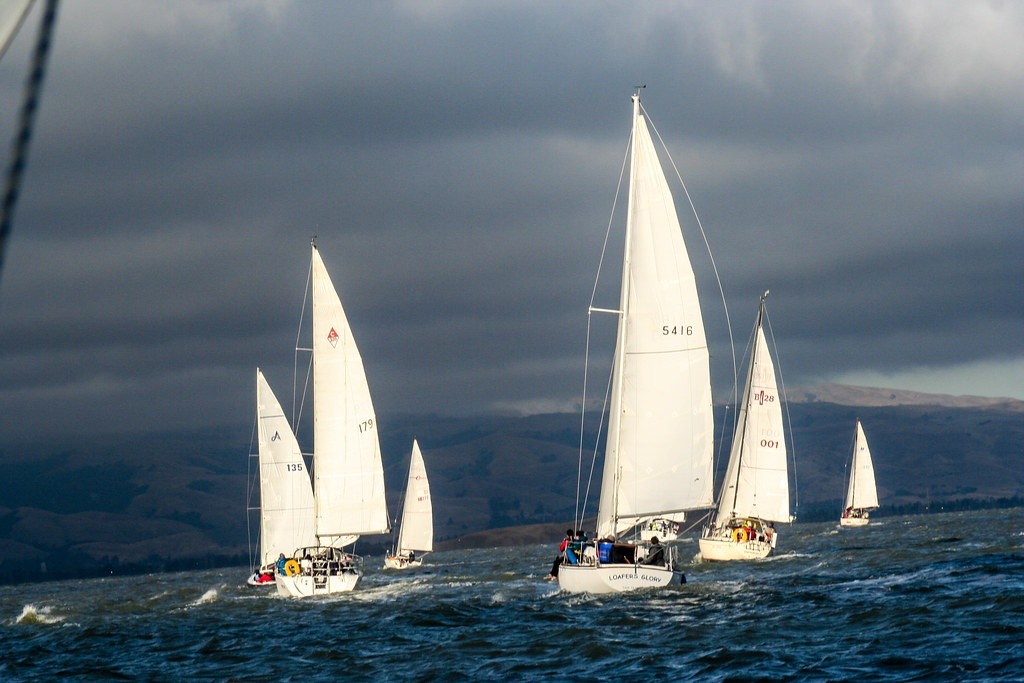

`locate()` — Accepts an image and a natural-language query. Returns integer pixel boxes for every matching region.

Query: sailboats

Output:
[839,417,881,528]
[697,290,800,563]
[382,436,435,571]
[244,366,320,589]
[272,235,395,600]
[555,83,739,600]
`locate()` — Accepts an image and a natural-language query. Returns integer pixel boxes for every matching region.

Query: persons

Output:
[306,553,357,575]
[255,570,262,581]
[597,535,618,563]
[543,530,588,581]
[409,552,415,562]
[673,522,679,534]
[638,536,665,567]
[757,521,776,542]
[276,553,287,576]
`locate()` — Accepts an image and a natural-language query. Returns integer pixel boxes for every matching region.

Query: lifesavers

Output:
[733,528,747,542]
[285,560,300,576]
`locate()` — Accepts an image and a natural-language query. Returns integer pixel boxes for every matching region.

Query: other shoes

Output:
[543,574,553,580]
[548,576,558,583]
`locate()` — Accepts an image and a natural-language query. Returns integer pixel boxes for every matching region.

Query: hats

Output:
[608,535,615,542]
[304,554,312,560]
[279,553,285,558]
[651,536,659,544]
[575,530,584,537]
[566,528,574,537]
